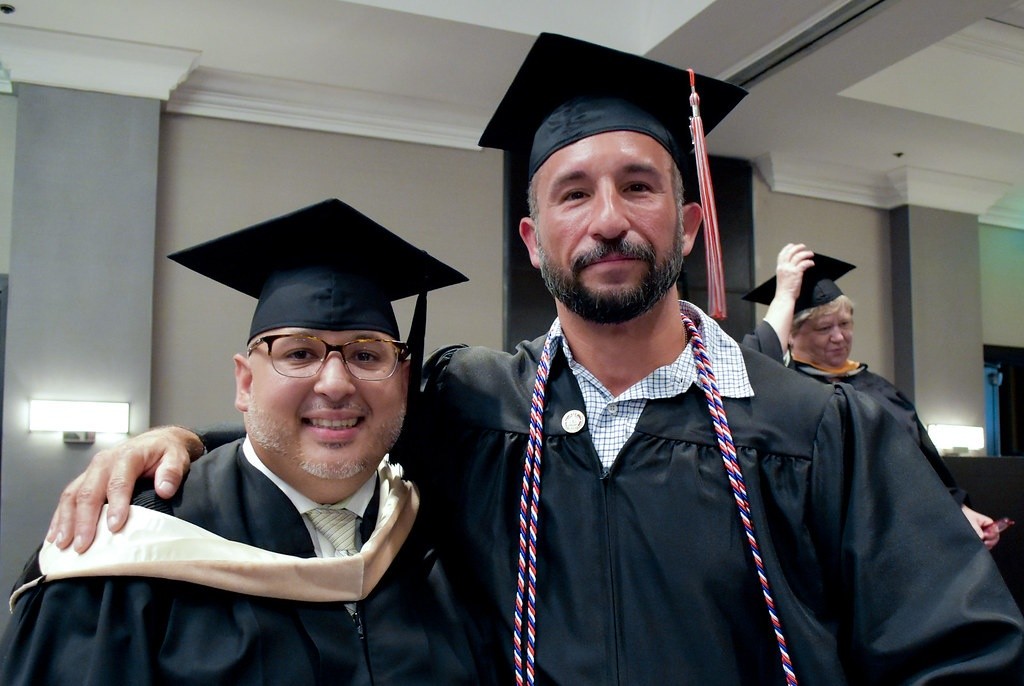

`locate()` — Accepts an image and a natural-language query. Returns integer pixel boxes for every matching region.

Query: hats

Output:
[166,197,470,480]
[739,252,857,314]
[476,32,751,320]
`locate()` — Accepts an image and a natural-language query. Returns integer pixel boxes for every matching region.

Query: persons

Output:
[0,197,514,686]
[739,243,999,551]
[46,32,1024,686]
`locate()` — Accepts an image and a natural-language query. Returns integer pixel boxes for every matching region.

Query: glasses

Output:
[245,333,409,382]
[981,518,1016,542]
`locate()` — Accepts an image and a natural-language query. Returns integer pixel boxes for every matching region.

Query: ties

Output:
[307,507,360,619]
